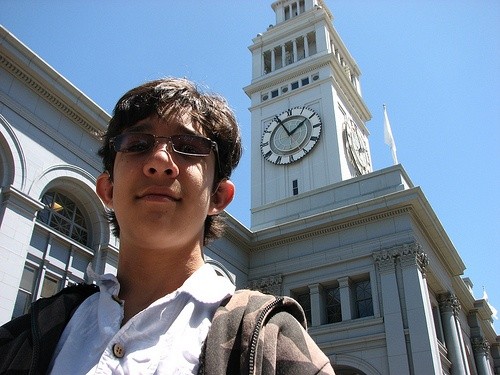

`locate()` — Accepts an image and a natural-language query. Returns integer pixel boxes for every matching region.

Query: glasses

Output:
[106,131,222,179]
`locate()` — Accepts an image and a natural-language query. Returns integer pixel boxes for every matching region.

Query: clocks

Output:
[345,120,372,176]
[260,106,322,165]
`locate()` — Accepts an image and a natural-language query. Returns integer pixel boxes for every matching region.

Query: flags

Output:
[384,105,398,166]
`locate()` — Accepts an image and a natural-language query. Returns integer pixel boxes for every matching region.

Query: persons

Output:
[0,76,336,375]
